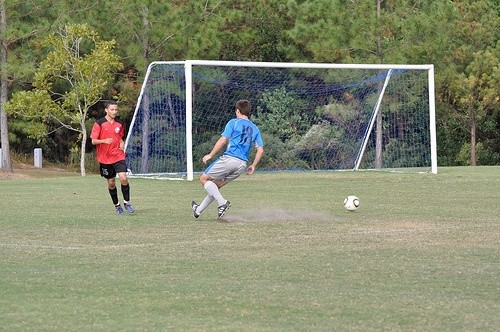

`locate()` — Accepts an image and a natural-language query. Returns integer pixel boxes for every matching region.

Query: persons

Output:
[90,100,134,215]
[191,99,264,219]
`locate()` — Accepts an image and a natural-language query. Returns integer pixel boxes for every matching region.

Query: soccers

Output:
[344,196,360,211]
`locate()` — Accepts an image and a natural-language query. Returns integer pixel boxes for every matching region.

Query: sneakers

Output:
[217,200,232,220]
[191,200,200,219]
[124,201,134,213]
[115,204,124,215]
